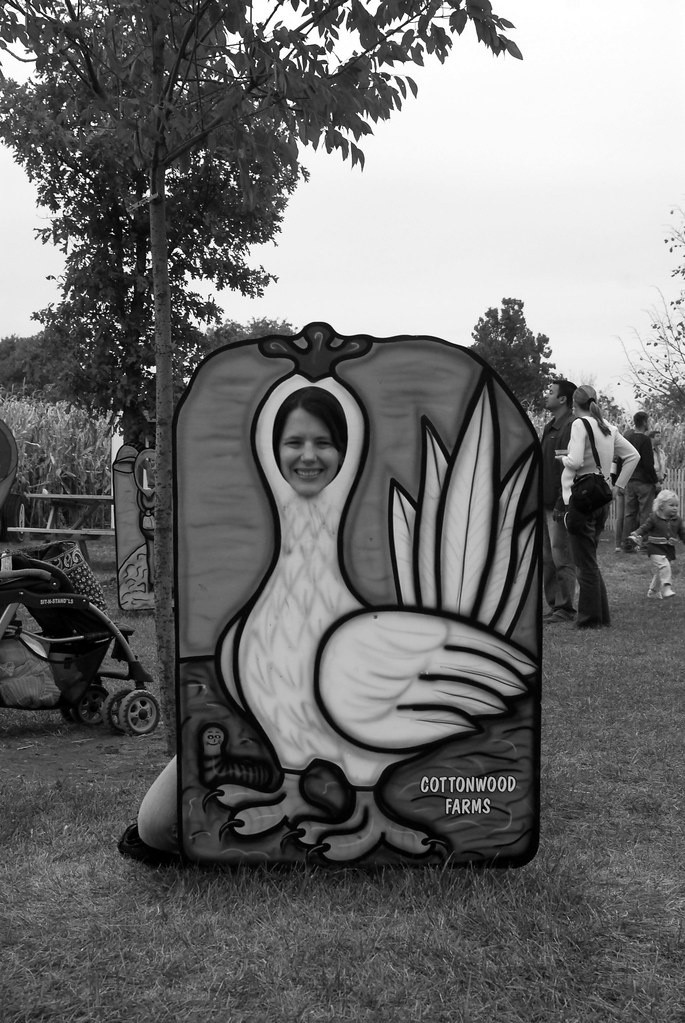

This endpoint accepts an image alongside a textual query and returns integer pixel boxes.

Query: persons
[137,386,348,850]
[610,411,668,553]
[625,489,685,600]
[540,380,641,630]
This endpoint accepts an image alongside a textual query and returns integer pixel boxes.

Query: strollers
[0,540,161,736]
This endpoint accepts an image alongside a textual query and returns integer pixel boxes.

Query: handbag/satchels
[571,473,613,514]
[13,540,108,617]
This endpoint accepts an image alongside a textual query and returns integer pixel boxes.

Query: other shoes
[564,623,610,630]
[663,586,676,597]
[543,612,576,623]
[647,590,663,599]
